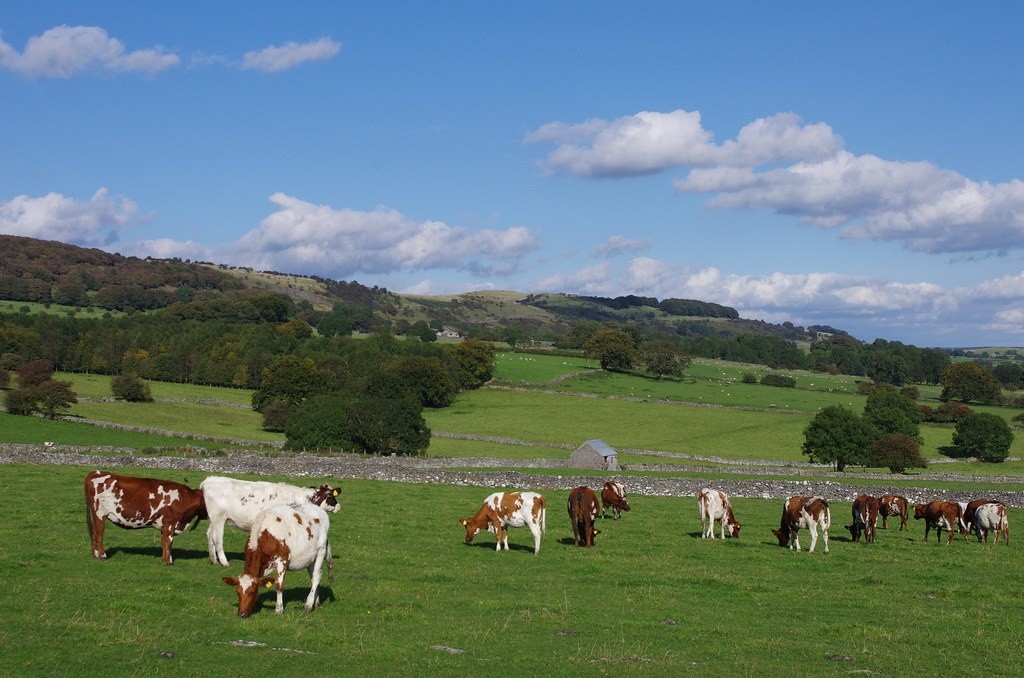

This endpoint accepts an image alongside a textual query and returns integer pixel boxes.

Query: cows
[876,494,909,532]
[601,480,631,521]
[459,491,546,556]
[770,495,831,554]
[843,495,880,545]
[696,488,743,540]
[82,469,343,619]
[567,485,602,547]
[911,499,1010,546]
